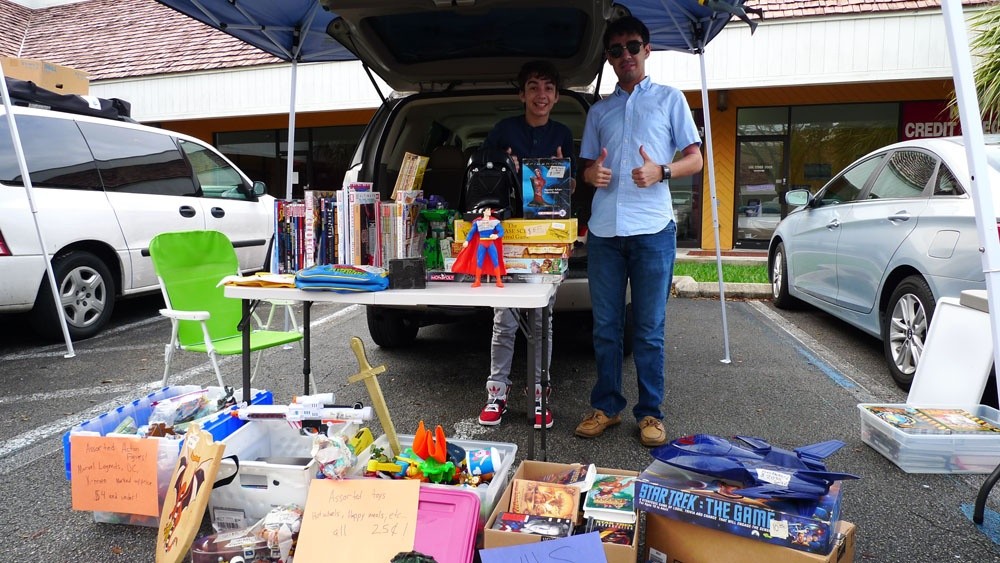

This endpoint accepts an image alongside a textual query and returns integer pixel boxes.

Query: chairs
[149,231,319,397]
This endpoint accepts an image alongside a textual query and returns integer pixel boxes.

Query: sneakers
[478,380,510,425]
[525,383,554,428]
[637,415,666,446]
[576,409,622,438]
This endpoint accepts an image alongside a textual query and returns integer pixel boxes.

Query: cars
[736,202,786,242]
[667,190,703,226]
[764,131,1000,392]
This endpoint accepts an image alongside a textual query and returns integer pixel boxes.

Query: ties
[606,40,649,58]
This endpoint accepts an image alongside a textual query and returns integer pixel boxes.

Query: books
[391,153,430,200]
[863,407,1000,472]
[273,183,424,273]
[492,463,636,545]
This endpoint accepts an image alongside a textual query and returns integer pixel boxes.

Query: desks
[223,279,558,462]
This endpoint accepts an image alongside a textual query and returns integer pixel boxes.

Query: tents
[161,0,762,364]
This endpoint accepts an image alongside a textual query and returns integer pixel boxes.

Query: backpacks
[294,263,390,291]
[458,148,522,217]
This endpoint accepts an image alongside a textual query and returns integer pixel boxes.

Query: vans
[0,106,277,342]
[315,1,633,349]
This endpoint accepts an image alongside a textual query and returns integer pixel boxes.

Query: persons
[477,60,578,429]
[575,15,703,449]
[492,515,563,536]
[416,202,462,271]
[451,208,507,287]
[529,169,546,205]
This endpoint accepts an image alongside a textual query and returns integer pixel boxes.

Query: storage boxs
[0,57,90,95]
[389,258,427,290]
[64,384,855,562]
[858,402,1000,475]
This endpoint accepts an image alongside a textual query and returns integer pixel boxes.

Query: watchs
[658,165,671,183]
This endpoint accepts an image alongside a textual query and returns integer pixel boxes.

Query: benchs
[426,142,597,224]
